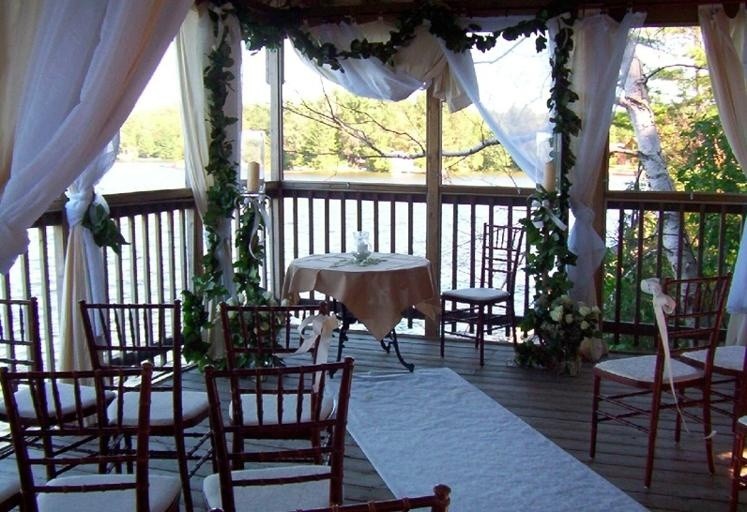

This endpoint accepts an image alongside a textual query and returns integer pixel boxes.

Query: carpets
[325,366,651,511]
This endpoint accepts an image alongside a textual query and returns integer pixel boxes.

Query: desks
[282,251,444,377]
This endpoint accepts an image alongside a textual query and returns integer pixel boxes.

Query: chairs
[0,299,116,481]
[1,360,184,512]
[80,299,217,512]
[202,357,353,512]
[590,270,735,488]
[440,222,524,366]
[1,461,41,512]
[300,483,450,511]
[674,344,747,443]
[728,414,747,511]
[222,302,332,468]
